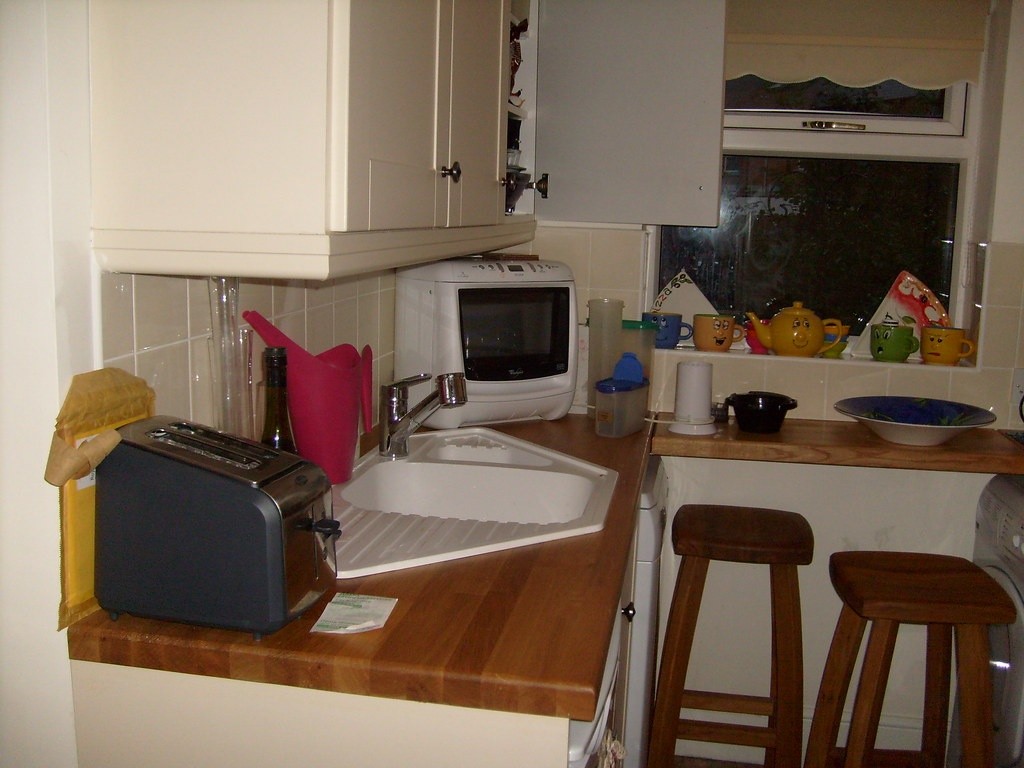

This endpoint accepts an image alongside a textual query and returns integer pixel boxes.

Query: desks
[652,411,1024,474]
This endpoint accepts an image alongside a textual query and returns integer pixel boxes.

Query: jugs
[241,310,373,485]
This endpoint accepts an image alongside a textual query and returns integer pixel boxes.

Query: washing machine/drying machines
[945,473,1024,768]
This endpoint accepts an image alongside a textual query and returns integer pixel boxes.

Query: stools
[642,502,813,768]
[803,551,1017,767]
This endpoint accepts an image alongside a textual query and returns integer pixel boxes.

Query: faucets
[377,370,468,456]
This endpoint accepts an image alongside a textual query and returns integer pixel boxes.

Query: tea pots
[746,301,843,357]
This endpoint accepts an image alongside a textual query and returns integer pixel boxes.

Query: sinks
[336,425,619,527]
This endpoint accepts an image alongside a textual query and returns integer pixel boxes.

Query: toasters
[94,417,341,641]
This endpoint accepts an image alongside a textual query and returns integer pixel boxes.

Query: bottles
[260,345,300,455]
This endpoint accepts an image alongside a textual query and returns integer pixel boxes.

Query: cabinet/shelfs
[84,0,733,279]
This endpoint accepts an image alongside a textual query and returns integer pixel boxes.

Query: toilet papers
[674,361,713,419]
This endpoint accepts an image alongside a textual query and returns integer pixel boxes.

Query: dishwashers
[622,454,668,766]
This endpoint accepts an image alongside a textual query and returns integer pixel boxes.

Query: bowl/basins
[506,173,531,212]
[835,396,998,446]
[726,391,798,434]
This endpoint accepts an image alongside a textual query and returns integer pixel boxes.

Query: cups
[744,320,771,354]
[824,326,850,358]
[507,149,521,173]
[693,314,744,352]
[921,326,975,365]
[871,324,919,362]
[587,299,623,417]
[642,311,693,350]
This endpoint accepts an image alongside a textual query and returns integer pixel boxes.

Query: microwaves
[392,258,578,429]
